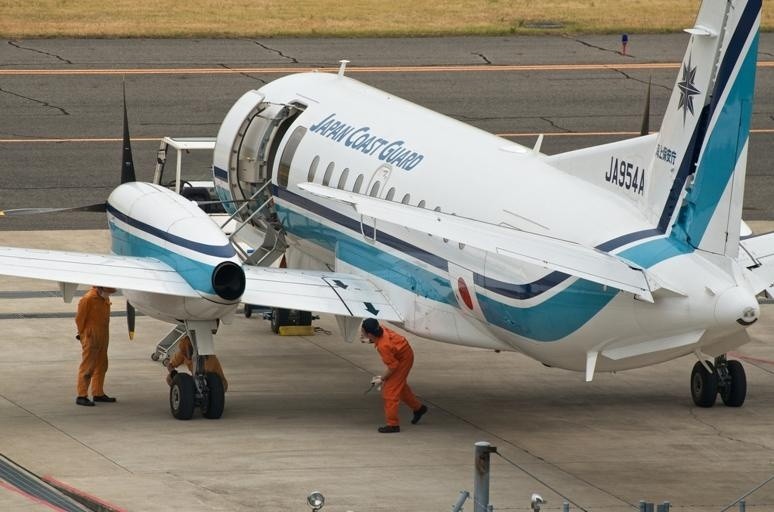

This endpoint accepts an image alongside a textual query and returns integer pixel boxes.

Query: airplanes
[0,0,773,422]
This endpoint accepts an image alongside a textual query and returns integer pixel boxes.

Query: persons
[360,318,428,434]
[75,285,116,407]
[166,335,228,394]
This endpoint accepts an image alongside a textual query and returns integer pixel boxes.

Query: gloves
[371,376,385,392]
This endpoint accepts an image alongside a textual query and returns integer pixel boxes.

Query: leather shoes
[411,405,426,424]
[378,425,400,432]
[93,395,115,402]
[77,397,94,406]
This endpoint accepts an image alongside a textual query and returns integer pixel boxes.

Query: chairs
[180,187,211,213]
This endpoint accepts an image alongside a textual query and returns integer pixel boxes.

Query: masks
[98,289,111,299]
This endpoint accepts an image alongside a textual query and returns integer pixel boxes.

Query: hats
[179,337,192,352]
[364,318,382,336]
[103,287,115,293]
